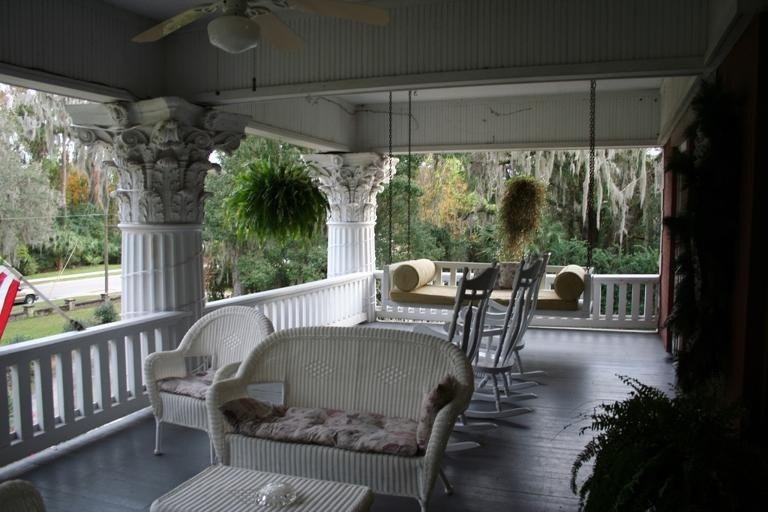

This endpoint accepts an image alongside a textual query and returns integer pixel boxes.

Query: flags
[0,265,21,340]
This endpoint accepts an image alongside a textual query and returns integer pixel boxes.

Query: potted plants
[497,175,548,289]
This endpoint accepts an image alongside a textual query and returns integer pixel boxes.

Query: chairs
[144,306,274,455]
[412,252,551,453]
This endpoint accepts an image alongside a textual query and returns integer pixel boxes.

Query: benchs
[205,327,474,512]
[382,260,595,318]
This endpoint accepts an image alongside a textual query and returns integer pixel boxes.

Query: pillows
[394,259,436,292]
[554,264,585,301]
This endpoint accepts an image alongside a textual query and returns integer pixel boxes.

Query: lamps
[208,16,259,95]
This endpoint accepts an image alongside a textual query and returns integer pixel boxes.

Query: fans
[129,0,390,49]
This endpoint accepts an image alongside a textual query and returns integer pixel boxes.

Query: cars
[14,286,39,304]
